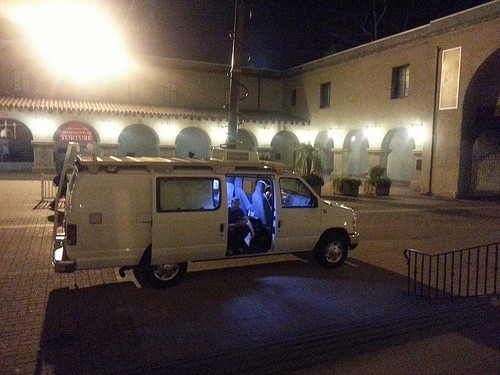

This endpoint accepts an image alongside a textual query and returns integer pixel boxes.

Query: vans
[47,141,358,289]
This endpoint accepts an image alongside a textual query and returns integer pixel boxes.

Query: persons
[227,197,255,255]
[49,170,69,208]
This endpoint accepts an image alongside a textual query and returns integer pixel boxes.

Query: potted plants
[332,177,363,196]
[290,142,324,198]
[364,164,392,196]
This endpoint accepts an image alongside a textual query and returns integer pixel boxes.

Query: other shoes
[227,248,244,254]
[49,204,54,208]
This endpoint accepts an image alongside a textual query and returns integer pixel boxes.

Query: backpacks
[52,174,60,186]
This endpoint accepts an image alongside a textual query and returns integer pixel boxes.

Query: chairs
[234,176,251,216]
[252,180,273,226]
